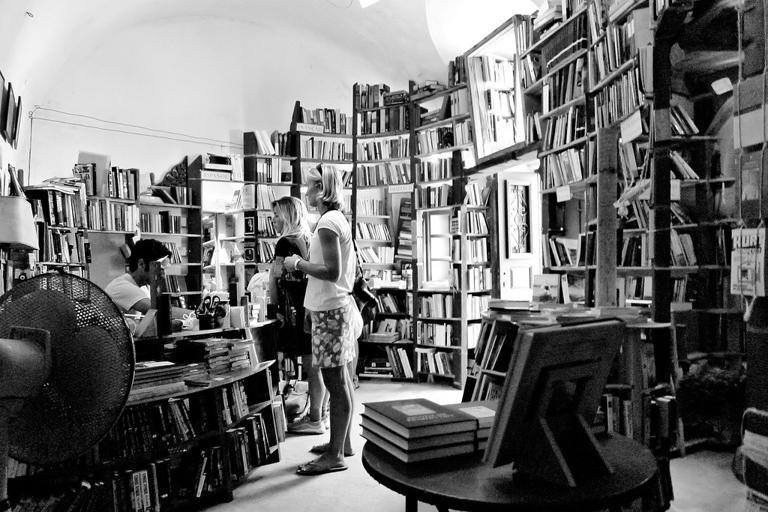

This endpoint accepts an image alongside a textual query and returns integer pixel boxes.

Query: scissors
[204,296,220,313]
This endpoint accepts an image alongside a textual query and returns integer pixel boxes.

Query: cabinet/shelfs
[0,0,767,512]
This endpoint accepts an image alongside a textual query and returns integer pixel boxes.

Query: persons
[268,162,362,475]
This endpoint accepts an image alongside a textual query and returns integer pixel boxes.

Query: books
[516,1,767,450]
[481,316,625,469]
[359,372,500,464]
[354,55,539,380]
[241,103,356,380]
[1,150,287,510]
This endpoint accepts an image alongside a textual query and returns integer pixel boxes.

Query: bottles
[156,293,172,335]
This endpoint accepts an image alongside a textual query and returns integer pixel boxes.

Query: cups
[230,305,245,329]
[199,312,215,330]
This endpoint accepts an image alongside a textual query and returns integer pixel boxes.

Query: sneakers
[287,416,326,435]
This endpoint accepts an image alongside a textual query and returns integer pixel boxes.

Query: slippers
[311,443,356,457]
[295,461,349,476]
